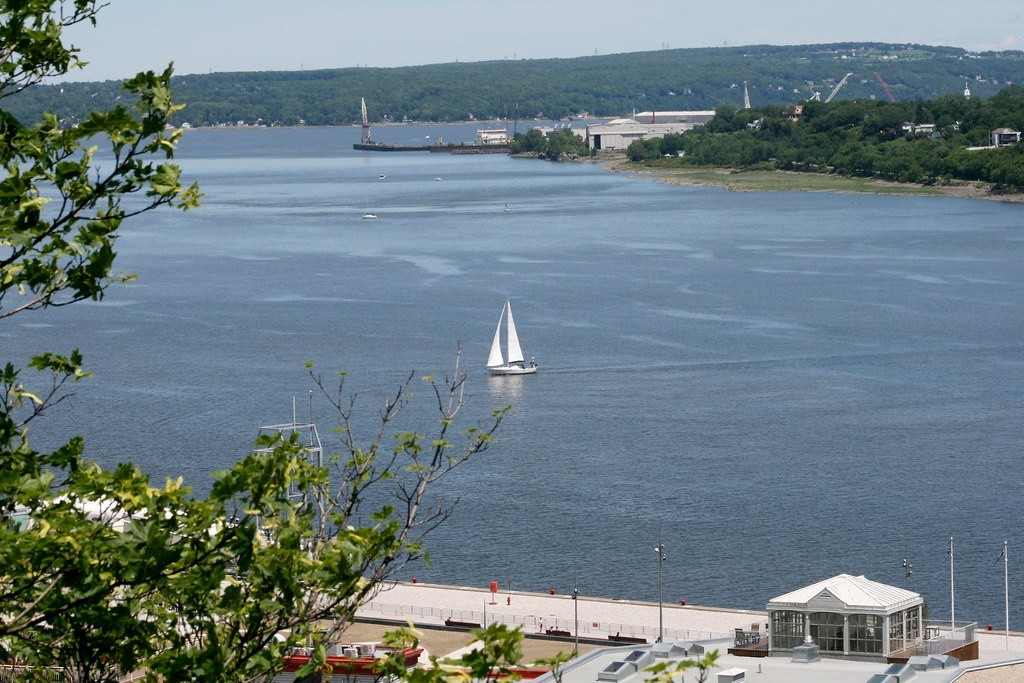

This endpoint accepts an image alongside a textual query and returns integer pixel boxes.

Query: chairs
[735,623,759,648]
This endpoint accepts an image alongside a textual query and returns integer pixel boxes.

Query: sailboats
[361,97,370,128]
[485,299,539,375]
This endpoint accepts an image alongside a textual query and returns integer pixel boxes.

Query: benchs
[546,630,572,636]
[445,621,481,628]
[608,636,646,645]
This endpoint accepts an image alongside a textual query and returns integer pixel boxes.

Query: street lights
[654,535,667,643]
[902,545,913,591]
[571,588,580,658]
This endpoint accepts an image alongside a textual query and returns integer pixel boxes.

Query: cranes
[873,72,897,103]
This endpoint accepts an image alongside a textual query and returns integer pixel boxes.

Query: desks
[925,626,939,639]
[744,631,759,644]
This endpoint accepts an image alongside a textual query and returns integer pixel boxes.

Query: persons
[538,617,560,633]
[447,617,452,621]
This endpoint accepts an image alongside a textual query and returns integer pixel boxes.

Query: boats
[362,213,377,219]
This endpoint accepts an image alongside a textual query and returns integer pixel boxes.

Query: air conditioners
[361,644,375,655]
[293,648,305,655]
[344,648,358,658]
